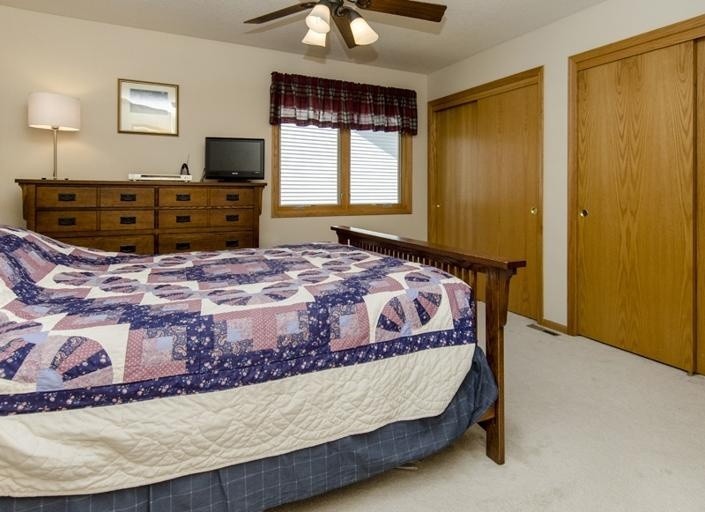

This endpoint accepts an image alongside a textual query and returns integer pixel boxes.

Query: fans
[244,0,448,49]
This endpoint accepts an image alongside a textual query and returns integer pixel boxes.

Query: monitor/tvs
[204,137,265,183]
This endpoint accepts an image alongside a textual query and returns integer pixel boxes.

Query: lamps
[301,0,379,47]
[27,92,80,181]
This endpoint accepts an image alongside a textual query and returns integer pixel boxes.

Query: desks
[15,179,268,255]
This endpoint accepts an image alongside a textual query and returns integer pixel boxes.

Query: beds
[0,225,527,512]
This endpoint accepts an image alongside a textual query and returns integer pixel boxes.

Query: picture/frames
[117,79,179,137]
